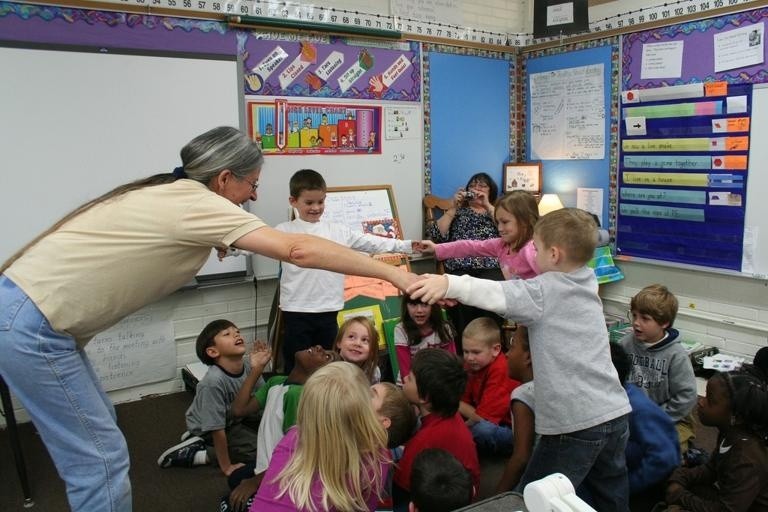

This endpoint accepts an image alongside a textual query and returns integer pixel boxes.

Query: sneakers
[157,432,205,467]
[686,447,710,466]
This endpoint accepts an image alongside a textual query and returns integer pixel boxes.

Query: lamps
[537,194,565,216]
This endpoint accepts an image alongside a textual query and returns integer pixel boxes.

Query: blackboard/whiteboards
[1,46,247,285]
[237,96,425,286]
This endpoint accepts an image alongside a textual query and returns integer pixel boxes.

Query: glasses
[240,179,259,193]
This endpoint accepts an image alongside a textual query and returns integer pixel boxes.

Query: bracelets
[446,210,456,218]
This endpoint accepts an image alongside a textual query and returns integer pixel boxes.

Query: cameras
[463,191,476,201]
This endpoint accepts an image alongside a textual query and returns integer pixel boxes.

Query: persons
[157,320,268,468]
[215,168,432,376]
[607,340,680,510]
[411,190,540,326]
[407,206,631,511]
[656,370,767,512]
[426,172,508,351]
[0,126,428,512]
[220,290,536,512]
[619,284,697,462]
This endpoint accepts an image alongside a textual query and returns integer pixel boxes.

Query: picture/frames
[504,164,542,192]
